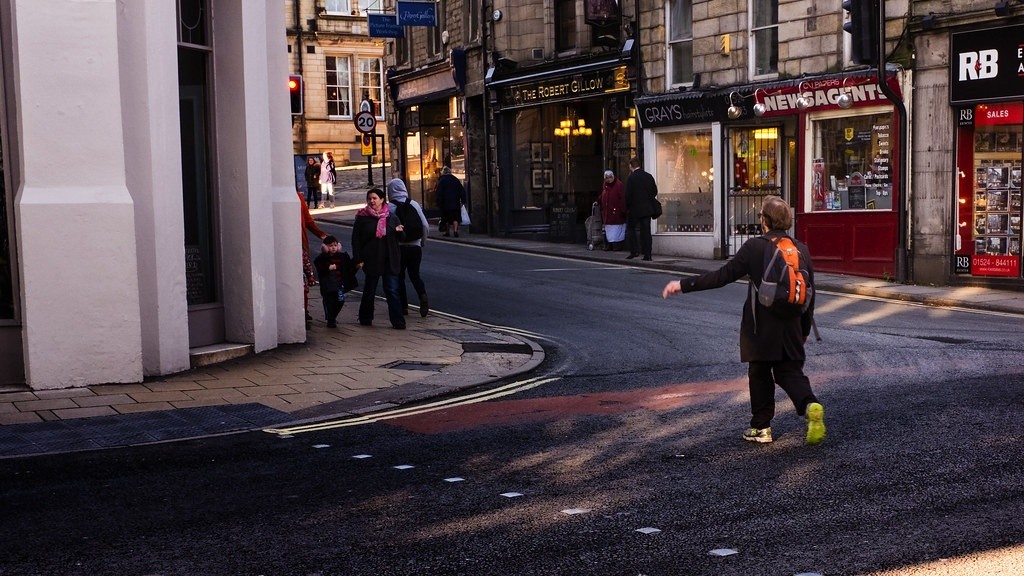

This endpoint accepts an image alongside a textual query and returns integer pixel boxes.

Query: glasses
[758,213,763,220]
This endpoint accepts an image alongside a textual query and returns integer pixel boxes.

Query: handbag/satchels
[459,204,472,227]
[649,197,663,219]
[438,219,447,232]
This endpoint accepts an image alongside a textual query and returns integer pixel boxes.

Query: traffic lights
[289,74,304,116]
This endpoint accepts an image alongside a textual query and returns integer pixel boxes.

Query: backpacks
[753,232,813,319]
[389,198,423,243]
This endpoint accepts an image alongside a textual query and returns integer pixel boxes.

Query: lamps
[498,57,519,68]
[598,34,617,49]
[753,88,782,117]
[728,91,764,120]
[554,106,592,137]
[837,77,857,109]
[755,128,777,139]
[796,81,824,109]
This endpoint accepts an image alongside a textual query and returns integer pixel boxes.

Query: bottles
[814,174,823,209]
[825,182,846,210]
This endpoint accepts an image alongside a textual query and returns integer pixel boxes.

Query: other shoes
[318,204,325,209]
[314,204,318,209]
[641,255,652,261]
[403,308,409,315]
[392,320,406,330]
[360,318,373,326]
[305,312,312,320]
[626,250,639,259]
[613,245,622,252]
[327,317,338,328]
[420,293,428,317]
[605,245,612,250]
[454,232,458,237]
[330,204,336,208]
[443,233,450,237]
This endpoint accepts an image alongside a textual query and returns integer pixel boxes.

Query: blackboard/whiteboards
[550,206,578,242]
[848,185,867,209]
[185,245,207,298]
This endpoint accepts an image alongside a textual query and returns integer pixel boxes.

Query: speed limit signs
[354,110,377,133]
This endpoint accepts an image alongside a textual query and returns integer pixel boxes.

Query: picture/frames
[530,167,554,191]
[529,140,553,164]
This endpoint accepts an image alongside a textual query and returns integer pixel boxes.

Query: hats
[442,166,451,175]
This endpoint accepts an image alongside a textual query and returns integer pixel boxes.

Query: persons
[595,170,626,250]
[305,151,336,208]
[387,177,430,318]
[297,192,328,320]
[832,192,841,208]
[352,188,406,330]
[314,235,363,328]
[624,157,658,261]
[436,166,466,238]
[663,196,825,444]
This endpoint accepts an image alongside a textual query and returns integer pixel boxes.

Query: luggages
[584,201,605,250]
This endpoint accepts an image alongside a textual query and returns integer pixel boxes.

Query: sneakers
[804,402,826,446]
[743,427,772,443]
